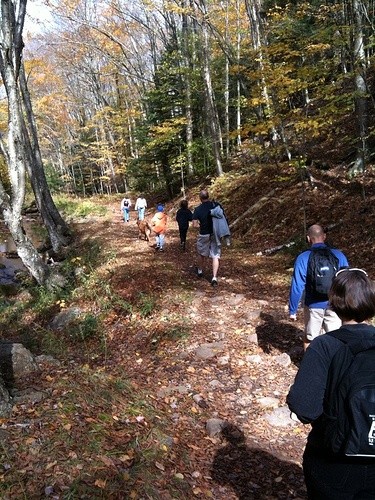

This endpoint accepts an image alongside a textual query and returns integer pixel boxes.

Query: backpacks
[306,246,338,305]
[327,326,375,457]
[124,201,128,206]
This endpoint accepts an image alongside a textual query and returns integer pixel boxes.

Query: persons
[176,200,193,252]
[192,190,226,286]
[289,223,349,351]
[150,205,168,251]
[135,195,147,221]
[121,195,131,222]
[286,267,375,500]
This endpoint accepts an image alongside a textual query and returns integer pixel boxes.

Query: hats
[158,206,163,211]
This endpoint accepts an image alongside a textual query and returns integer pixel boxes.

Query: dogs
[136,219,152,242]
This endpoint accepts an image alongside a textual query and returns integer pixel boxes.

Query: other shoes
[211,277,217,285]
[197,268,202,276]
[156,244,160,248]
[160,248,163,252]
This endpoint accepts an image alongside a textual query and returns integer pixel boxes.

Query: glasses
[333,265,368,278]
